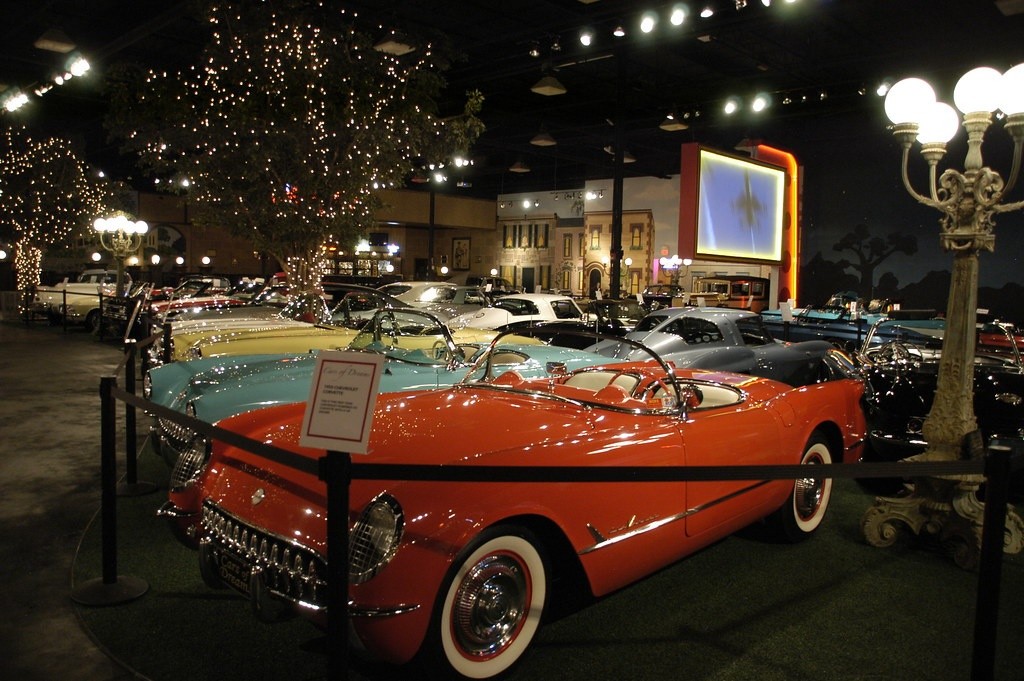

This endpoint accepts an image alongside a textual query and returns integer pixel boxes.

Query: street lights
[427,152,469,283]
[94,216,148,301]
[863,63,1024,570]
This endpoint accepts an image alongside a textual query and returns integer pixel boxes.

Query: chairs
[444,344,482,363]
[492,349,530,363]
[641,378,743,409]
[562,369,641,399]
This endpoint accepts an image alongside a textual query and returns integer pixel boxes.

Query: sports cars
[155,328,867,681]
[32,271,1024,488]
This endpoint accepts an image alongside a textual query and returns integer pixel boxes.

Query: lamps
[2,42,95,118]
[665,82,894,119]
[522,0,804,60]
